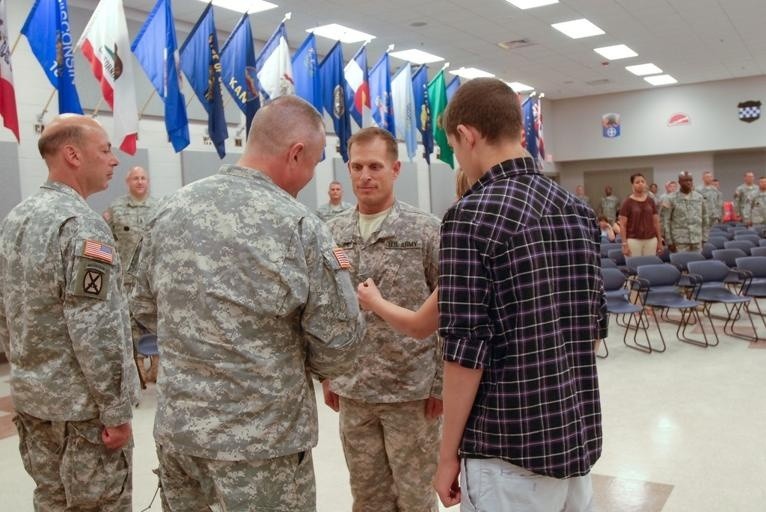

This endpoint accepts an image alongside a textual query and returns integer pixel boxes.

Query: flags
[131,4,192,154]
[218,10,261,140]
[178,3,229,159]
[291,32,461,170]
[255,22,297,103]
[1,6,20,144]
[77,2,140,154]
[20,3,85,117]
[520,96,545,173]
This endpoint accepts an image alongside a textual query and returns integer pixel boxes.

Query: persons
[316,179,349,219]
[120,92,353,511]
[575,156,764,316]
[311,126,452,511]
[105,167,158,371]
[450,163,470,202]
[432,74,608,510]
[1,114,142,511]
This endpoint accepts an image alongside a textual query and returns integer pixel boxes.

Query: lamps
[593,222,766,359]
